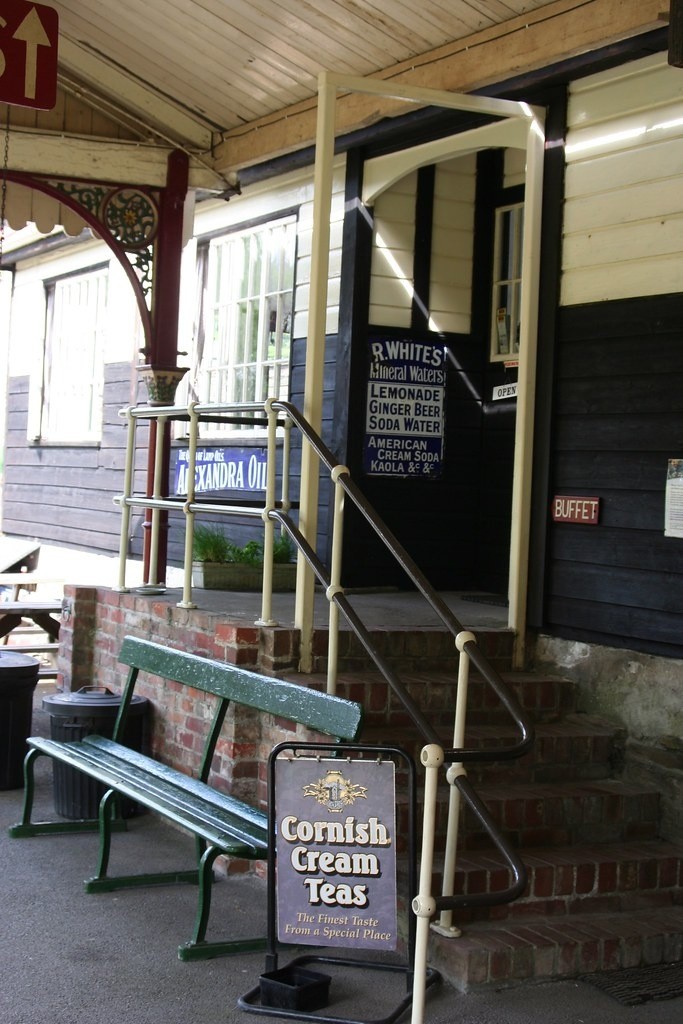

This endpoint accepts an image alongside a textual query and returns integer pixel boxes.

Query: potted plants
[190,520,298,593]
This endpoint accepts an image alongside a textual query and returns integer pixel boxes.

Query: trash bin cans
[0,651,41,793]
[40,683,150,820]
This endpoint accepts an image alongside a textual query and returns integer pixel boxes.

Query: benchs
[7,636,363,961]
